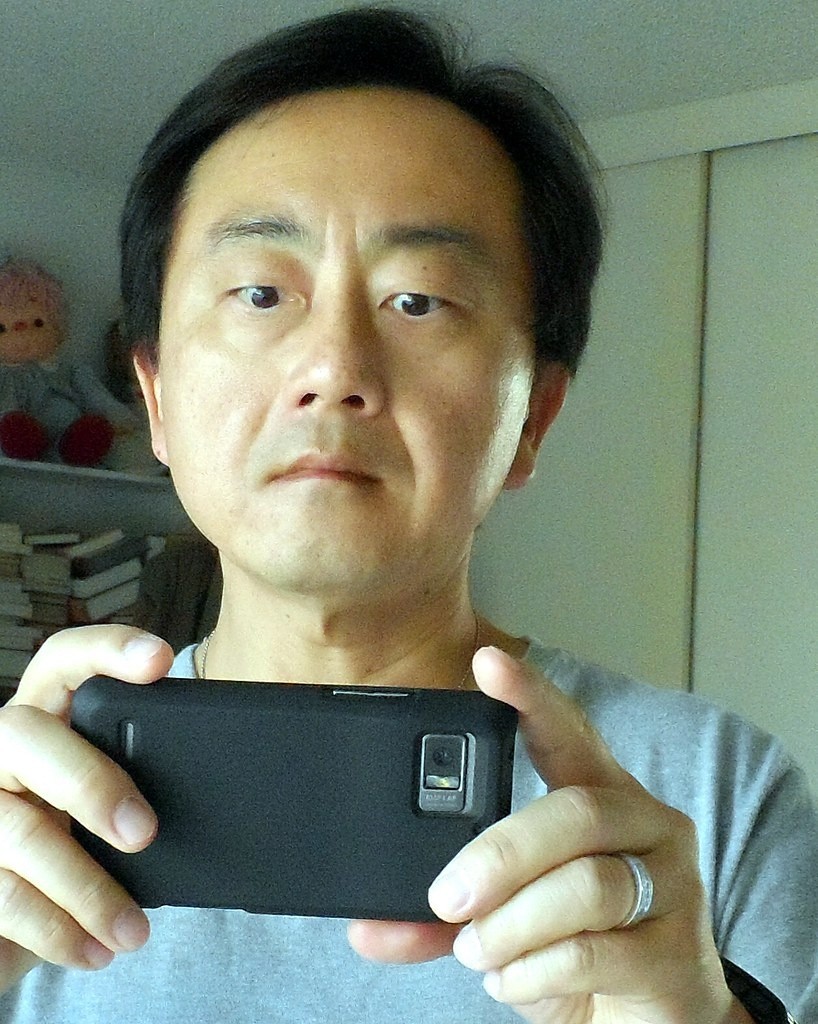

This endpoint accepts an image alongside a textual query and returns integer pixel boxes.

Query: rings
[612,851,653,930]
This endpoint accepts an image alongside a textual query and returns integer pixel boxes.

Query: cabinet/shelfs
[463,78,818,813]
[0,453,216,699]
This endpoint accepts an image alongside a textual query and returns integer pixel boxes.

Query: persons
[0,5,818,1024]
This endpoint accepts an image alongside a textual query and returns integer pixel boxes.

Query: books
[0,522,204,680]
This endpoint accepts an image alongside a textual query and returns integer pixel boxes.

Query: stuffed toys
[0,256,164,473]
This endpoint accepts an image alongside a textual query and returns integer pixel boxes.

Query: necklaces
[200,607,480,691]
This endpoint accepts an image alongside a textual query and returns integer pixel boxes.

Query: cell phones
[69,676,521,922]
